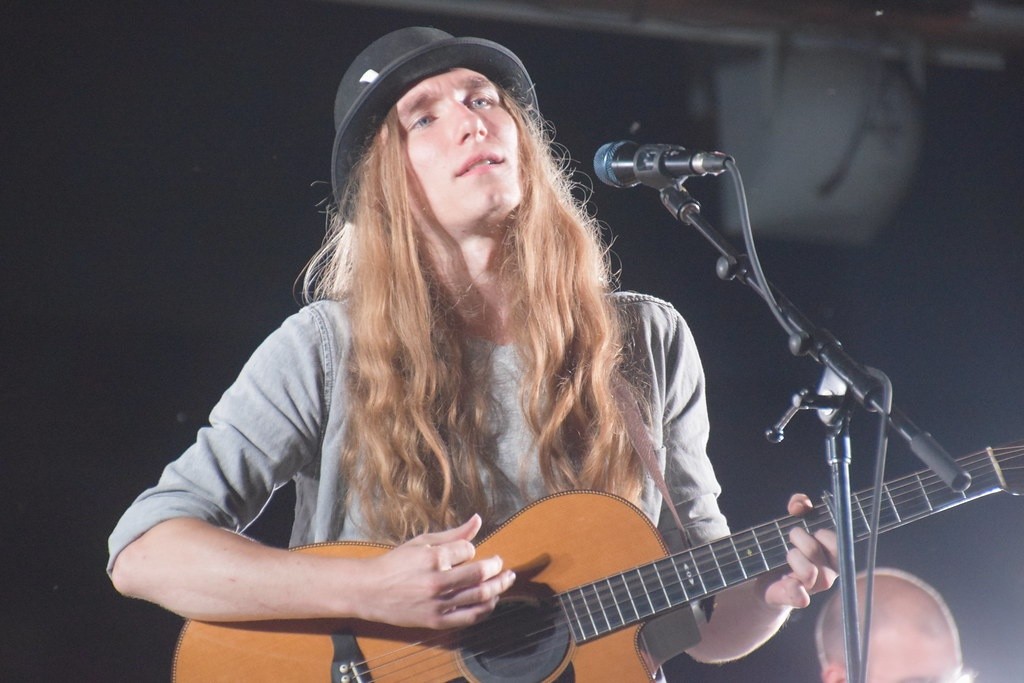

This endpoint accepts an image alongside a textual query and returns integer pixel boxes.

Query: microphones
[593,140,736,190]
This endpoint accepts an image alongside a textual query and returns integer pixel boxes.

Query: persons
[106,30,840,682]
[816,566,967,683]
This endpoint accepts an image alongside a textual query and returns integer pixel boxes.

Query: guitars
[170,441,1023,683]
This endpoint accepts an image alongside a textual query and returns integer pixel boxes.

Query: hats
[330,25,540,224]
